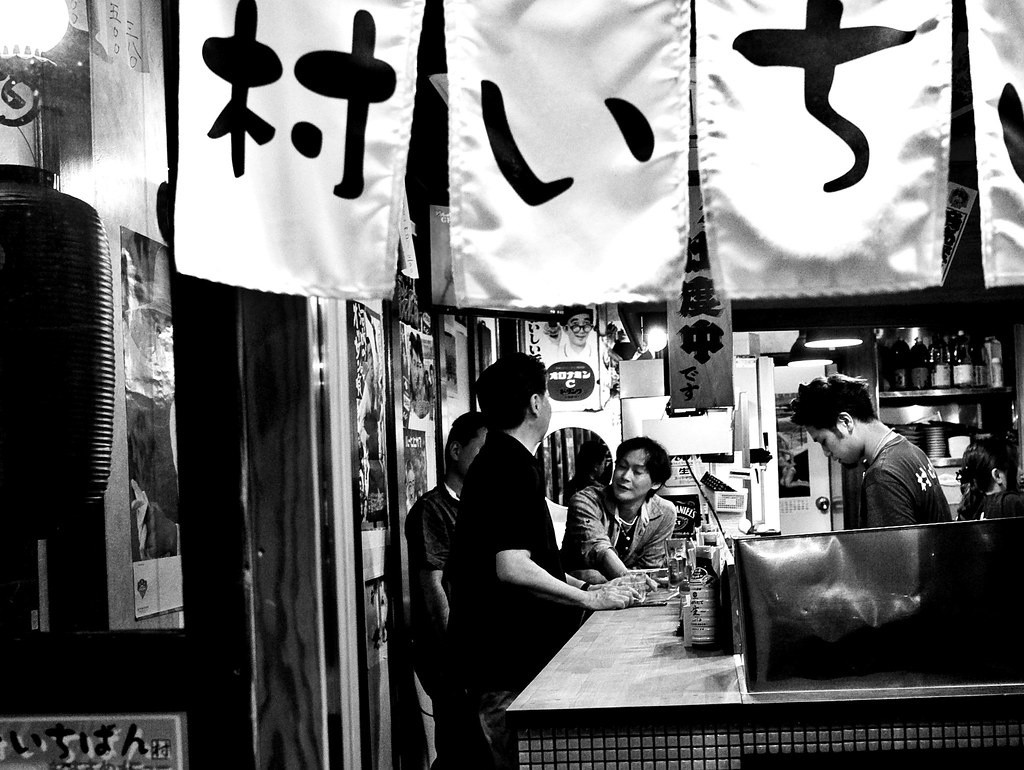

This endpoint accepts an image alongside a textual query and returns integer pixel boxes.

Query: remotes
[700,471,737,492]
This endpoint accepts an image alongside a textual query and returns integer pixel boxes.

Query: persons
[355,303,610,532]
[788,375,951,528]
[446,352,646,770]
[559,436,677,624]
[404,412,488,770]
[956,435,1024,521]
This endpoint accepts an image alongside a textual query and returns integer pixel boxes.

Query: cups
[620,571,646,604]
[664,538,687,593]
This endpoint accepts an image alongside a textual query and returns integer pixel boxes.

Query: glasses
[568,323,594,334]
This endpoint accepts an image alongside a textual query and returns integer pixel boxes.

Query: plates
[897,430,922,450]
[925,424,947,459]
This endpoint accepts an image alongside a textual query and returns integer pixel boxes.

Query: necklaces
[870,427,895,466]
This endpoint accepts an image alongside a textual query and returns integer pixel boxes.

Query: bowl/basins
[949,436,972,457]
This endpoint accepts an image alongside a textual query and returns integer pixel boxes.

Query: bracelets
[580,581,591,591]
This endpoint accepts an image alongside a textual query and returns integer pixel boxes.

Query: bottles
[889,330,1007,391]
[679,578,691,620]
[702,523,716,534]
[703,534,716,547]
[690,547,722,651]
